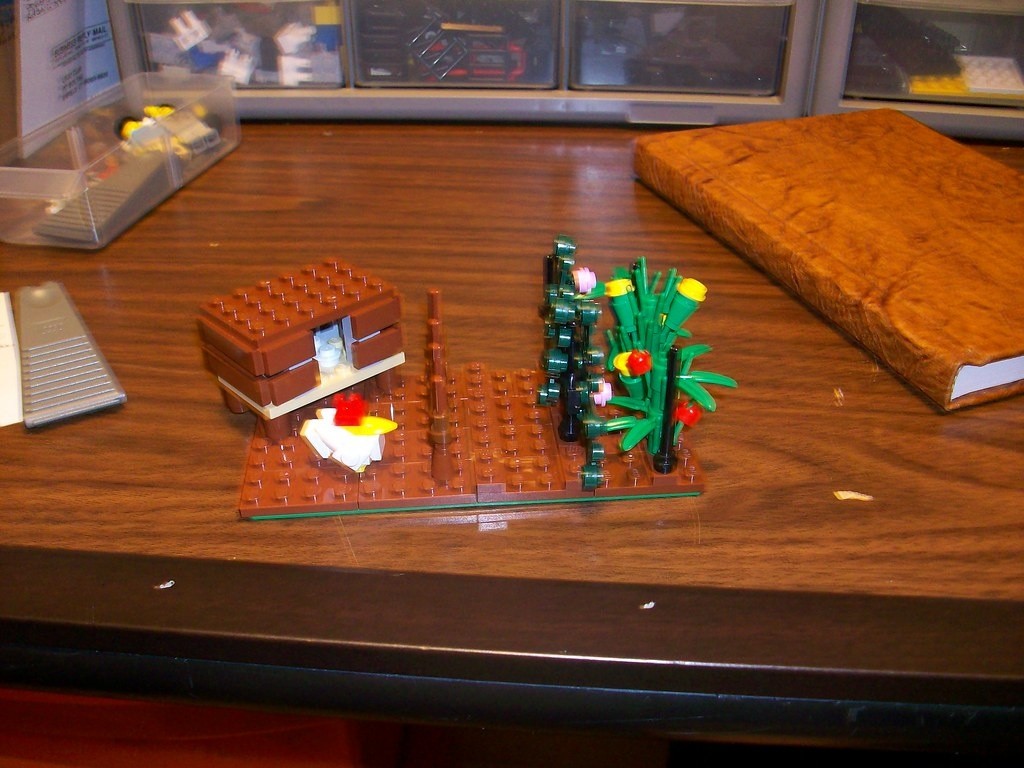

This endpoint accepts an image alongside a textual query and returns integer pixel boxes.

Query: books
[632,108,1024,412]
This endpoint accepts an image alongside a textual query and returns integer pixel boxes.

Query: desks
[0,118,1024,681]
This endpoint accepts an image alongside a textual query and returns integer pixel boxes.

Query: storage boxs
[106,0,349,120]
[0,70,242,254]
[569,0,827,125]
[345,1,568,122]
[806,0,1024,142]
